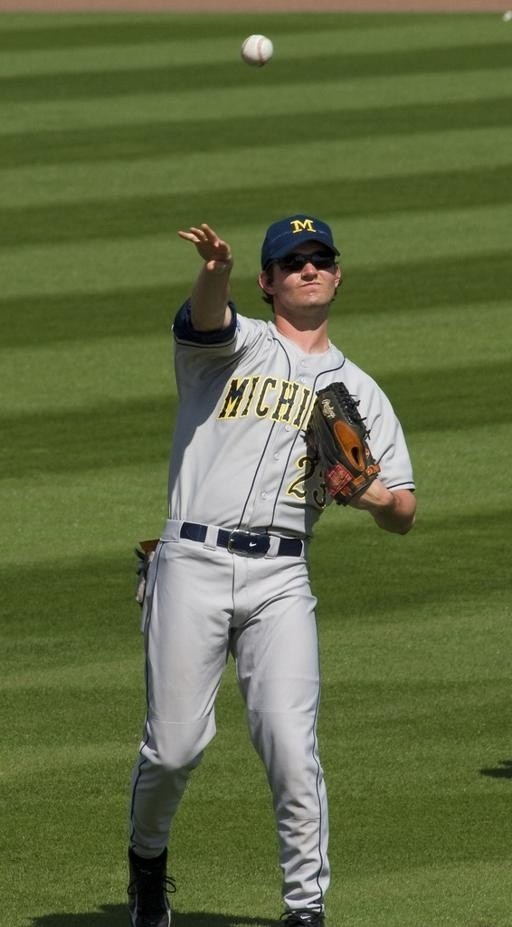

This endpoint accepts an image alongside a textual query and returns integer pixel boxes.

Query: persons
[118,214,422,927]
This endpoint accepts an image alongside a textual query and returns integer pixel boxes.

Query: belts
[180,521,302,560]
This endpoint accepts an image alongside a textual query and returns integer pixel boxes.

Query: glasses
[263,249,336,273]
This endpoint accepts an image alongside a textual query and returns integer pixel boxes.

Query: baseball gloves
[308,382,381,508]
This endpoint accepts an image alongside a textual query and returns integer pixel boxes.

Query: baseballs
[240,33,273,65]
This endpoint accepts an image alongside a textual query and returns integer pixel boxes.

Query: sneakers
[126,844,174,926]
[278,908,325,925]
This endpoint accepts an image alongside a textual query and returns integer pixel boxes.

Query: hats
[260,213,341,272]
